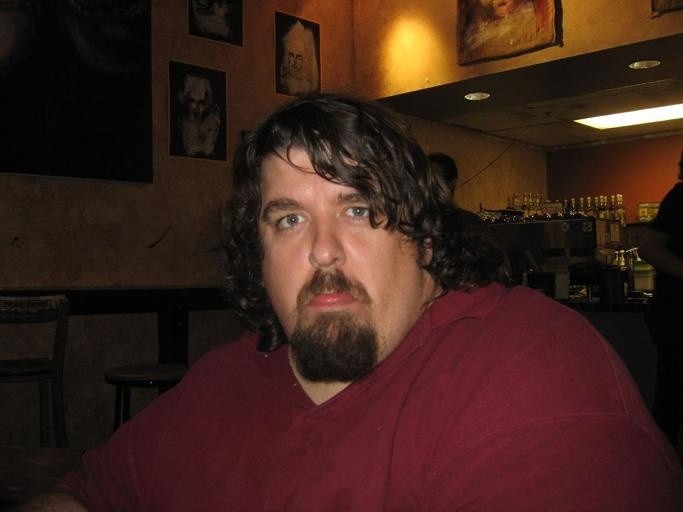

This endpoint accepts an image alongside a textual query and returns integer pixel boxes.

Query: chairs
[0,295,69,449]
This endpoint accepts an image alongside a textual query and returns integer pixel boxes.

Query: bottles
[510,191,627,228]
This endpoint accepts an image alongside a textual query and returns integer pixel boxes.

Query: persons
[626,153,681,455]
[7,88,681,512]
[411,149,492,246]
[174,75,222,156]
[278,19,318,95]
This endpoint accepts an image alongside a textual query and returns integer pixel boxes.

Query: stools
[104,365,181,433]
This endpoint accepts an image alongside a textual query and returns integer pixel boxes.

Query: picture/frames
[186,0,245,50]
[274,9,322,100]
[168,59,228,165]
